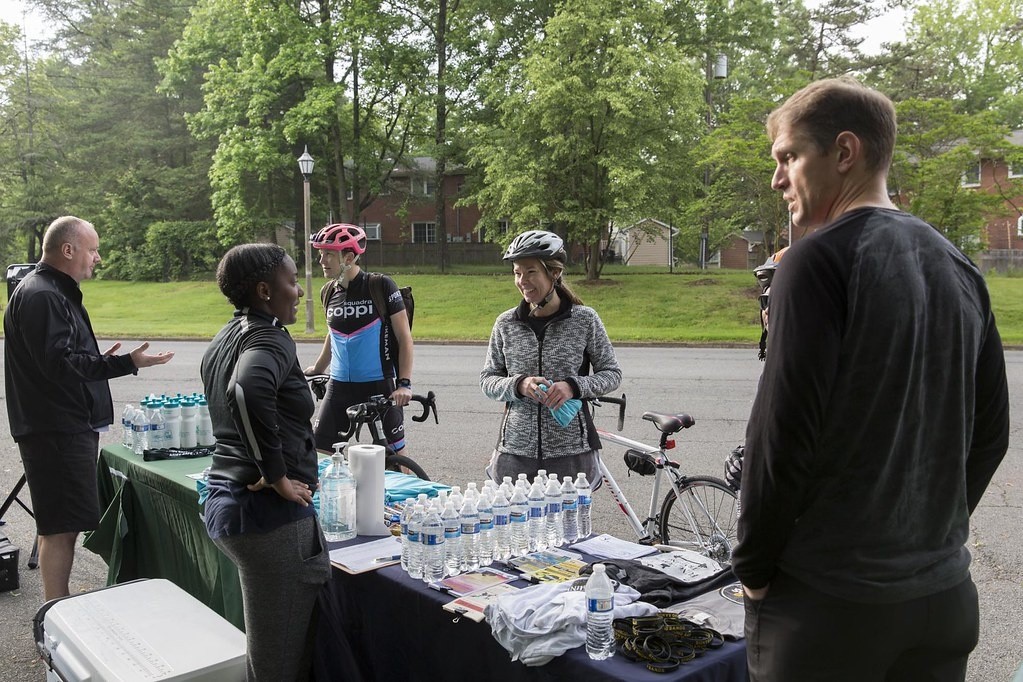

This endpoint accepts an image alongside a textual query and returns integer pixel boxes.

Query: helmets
[312,224,368,255]
[502,230,568,263]
[753,246,790,290]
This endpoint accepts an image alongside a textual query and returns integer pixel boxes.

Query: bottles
[585,564,616,660]
[121,392,216,455]
[401,470,591,581]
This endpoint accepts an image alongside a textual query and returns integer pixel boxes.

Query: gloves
[724,446,745,493]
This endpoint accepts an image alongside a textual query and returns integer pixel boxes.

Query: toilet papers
[348,444,393,536]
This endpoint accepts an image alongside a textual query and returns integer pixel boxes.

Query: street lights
[297,145,315,334]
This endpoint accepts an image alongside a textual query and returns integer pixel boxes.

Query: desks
[326,534,750,682]
[81,443,245,635]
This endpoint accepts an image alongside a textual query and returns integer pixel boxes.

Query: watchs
[396,378,411,386]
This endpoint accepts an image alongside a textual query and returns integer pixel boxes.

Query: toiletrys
[318,442,357,542]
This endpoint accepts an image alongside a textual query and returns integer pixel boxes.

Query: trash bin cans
[6,263,37,304]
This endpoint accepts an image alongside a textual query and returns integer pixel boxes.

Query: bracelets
[398,384,411,389]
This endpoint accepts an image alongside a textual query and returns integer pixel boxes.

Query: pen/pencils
[375,554,401,563]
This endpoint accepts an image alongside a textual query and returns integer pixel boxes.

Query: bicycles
[337,392,439,482]
[587,393,739,570]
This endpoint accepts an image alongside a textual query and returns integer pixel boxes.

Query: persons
[480,230,622,489]
[724,75,1012,682]
[203,243,363,681]
[3,214,176,602]
[301,223,412,476]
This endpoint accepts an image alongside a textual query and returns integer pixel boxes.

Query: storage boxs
[33,577,246,682]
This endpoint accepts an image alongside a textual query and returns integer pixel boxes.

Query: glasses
[757,294,769,311]
